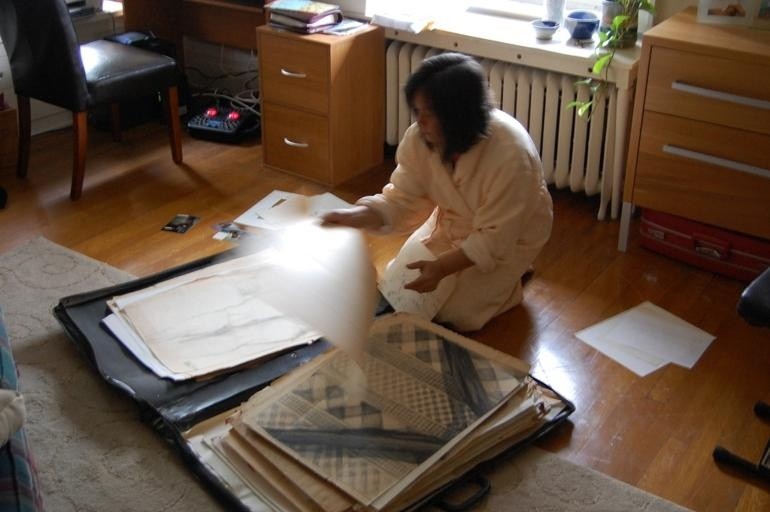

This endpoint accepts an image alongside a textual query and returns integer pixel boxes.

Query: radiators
[384,41,618,224]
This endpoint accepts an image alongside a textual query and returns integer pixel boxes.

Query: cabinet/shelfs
[613,0,769,259]
[257,18,386,188]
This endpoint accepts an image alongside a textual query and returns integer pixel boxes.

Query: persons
[319,52,554,334]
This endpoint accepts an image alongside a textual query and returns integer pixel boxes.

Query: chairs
[0,1,184,200]
[712,267,769,490]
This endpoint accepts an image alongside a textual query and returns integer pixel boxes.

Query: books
[263,8,343,35]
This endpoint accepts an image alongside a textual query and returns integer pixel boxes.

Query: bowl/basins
[532,19,560,39]
[564,10,600,40]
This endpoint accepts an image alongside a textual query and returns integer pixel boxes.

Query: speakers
[104,32,159,132]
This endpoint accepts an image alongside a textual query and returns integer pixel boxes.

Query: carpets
[0,233,693,512]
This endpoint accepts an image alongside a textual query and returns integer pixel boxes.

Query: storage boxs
[639,210,770,285]
